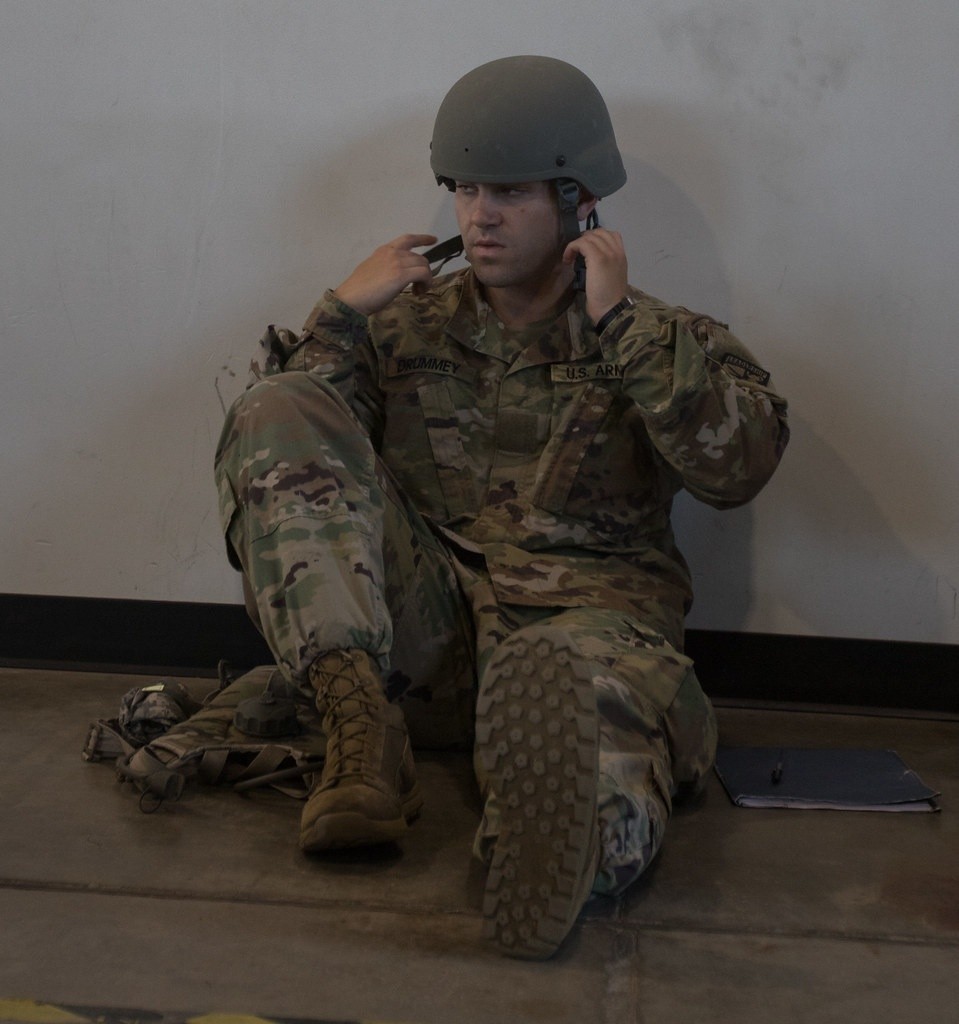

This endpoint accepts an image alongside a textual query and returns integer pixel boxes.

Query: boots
[296,646,424,855]
[473,627,599,962]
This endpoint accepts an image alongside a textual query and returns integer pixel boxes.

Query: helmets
[430,55,627,196]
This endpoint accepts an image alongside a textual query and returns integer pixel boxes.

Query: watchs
[594,295,643,337]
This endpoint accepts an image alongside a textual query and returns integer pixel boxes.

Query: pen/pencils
[772,761,784,782]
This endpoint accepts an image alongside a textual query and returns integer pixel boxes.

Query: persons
[212,54,789,958]
